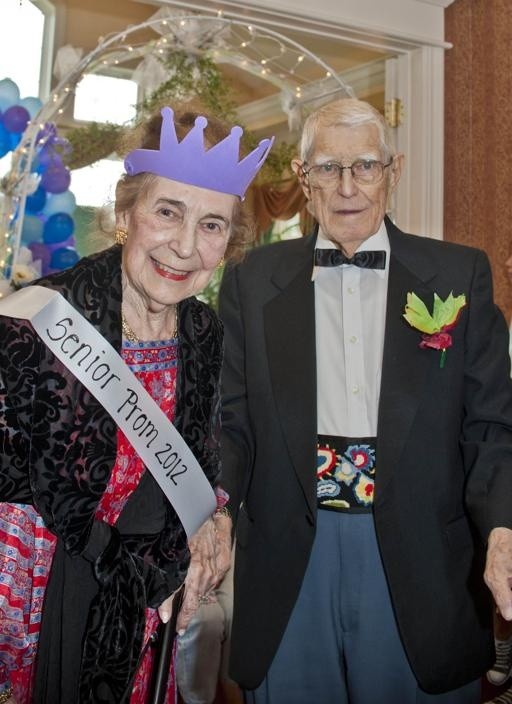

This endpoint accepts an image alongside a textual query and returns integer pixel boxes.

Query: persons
[1,104,277,703]
[211,94,511,701]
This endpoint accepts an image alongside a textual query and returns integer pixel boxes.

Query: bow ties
[315,249,387,270]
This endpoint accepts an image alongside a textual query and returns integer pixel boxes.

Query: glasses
[300,160,392,186]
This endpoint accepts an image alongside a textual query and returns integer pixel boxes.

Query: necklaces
[115,305,179,344]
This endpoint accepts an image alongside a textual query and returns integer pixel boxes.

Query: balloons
[1,75,82,280]
